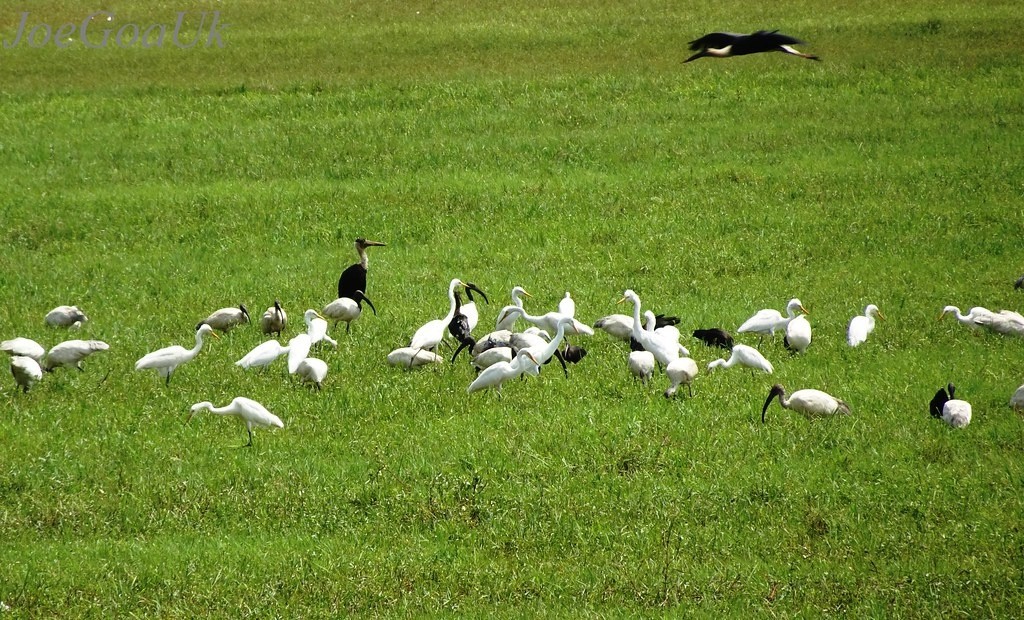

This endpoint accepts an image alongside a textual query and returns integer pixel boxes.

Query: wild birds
[197,303,334,392]
[762,383,847,427]
[594,290,697,402]
[682,28,821,66]
[938,305,1024,341]
[927,382,974,428]
[691,298,812,378]
[45,306,87,331]
[388,278,594,400]
[0,336,111,391]
[848,304,887,349]
[132,323,219,390]
[184,395,282,448]
[325,236,388,333]
